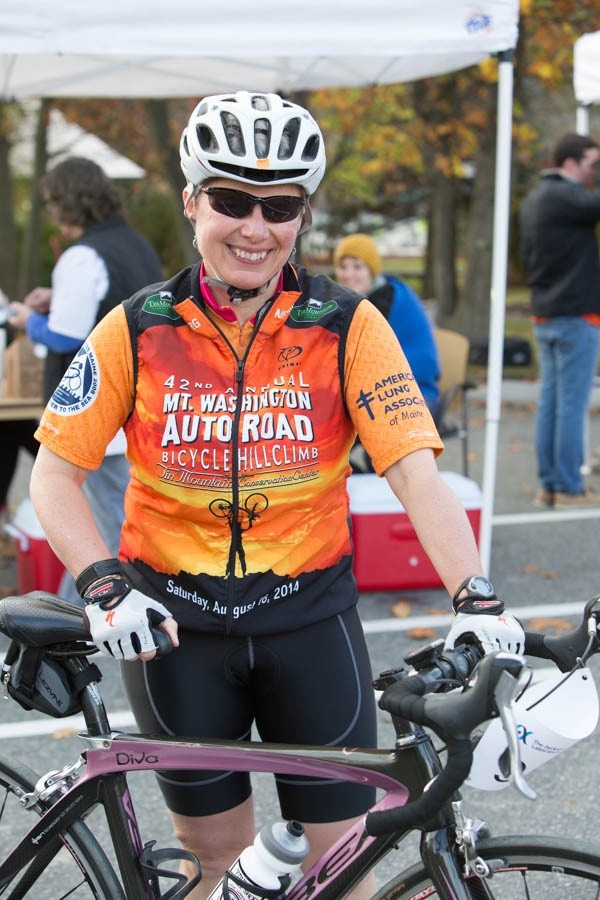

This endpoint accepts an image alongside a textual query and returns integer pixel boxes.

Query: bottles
[206,820,307,900]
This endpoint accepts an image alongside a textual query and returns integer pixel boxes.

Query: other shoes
[533,488,600,510]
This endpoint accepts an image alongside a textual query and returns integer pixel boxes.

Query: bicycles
[1,587,600,900]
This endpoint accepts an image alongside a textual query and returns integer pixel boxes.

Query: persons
[518,129,600,508]
[29,92,526,900]
[9,158,168,610]
[332,233,441,472]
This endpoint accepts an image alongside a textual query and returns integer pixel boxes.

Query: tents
[0,0,517,578]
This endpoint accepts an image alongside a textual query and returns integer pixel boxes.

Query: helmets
[178,92,327,196]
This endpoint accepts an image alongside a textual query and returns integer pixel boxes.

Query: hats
[334,234,381,280]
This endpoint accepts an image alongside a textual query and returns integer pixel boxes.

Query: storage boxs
[348,474,482,589]
[5,500,67,598]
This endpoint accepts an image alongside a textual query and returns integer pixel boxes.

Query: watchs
[451,575,498,615]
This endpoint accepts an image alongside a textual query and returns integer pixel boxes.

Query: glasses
[197,188,306,223]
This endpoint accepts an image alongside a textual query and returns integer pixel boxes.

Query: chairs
[429,329,475,476]
